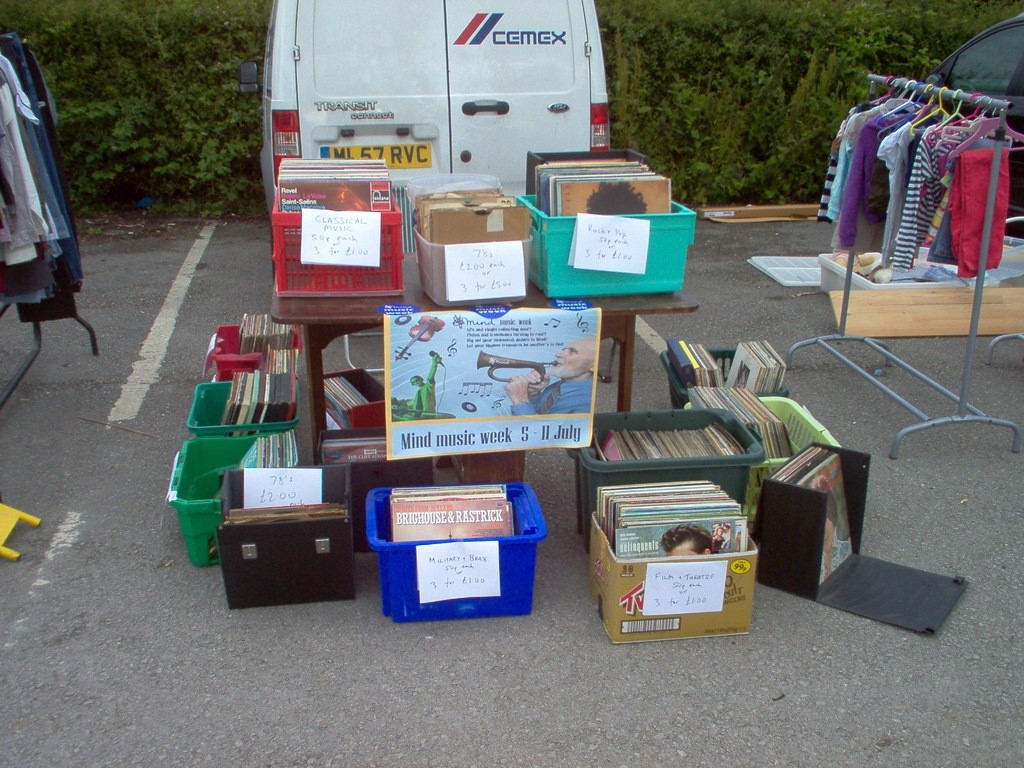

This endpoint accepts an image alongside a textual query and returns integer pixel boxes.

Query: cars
[903,13,1024,240]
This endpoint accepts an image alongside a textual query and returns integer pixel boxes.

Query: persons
[505,337,597,416]
[406,354,442,415]
[812,476,851,582]
[721,524,731,549]
[662,525,712,555]
[713,527,724,550]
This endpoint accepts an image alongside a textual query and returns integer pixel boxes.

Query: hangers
[847,75,1024,158]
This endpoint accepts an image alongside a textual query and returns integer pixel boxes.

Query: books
[666,337,787,392]
[415,188,532,245]
[209,311,301,561]
[687,385,794,458]
[597,479,749,559]
[323,376,370,430]
[534,158,671,217]
[592,424,747,463]
[322,436,387,463]
[279,158,392,215]
[772,446,853,584]
[387,484,512,541]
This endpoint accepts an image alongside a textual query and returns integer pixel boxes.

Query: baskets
[682,395,841,522]
[270,186,405,299]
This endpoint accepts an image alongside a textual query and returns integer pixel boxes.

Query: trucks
[239,0,611,285]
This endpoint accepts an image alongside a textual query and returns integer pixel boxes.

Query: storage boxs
[515,194,697,300]
[658,349,789,408]
[818,237,1024,296]
[412,224,534,308]
[187,382,301,436]
[566,407,766,555]
[272,193,407,298]
[212,325,302,380]
[321,368,384,432]
[170,431,277,568]
[684,397,842,522]
[365,482,547,623]
[588,510,759,644]
